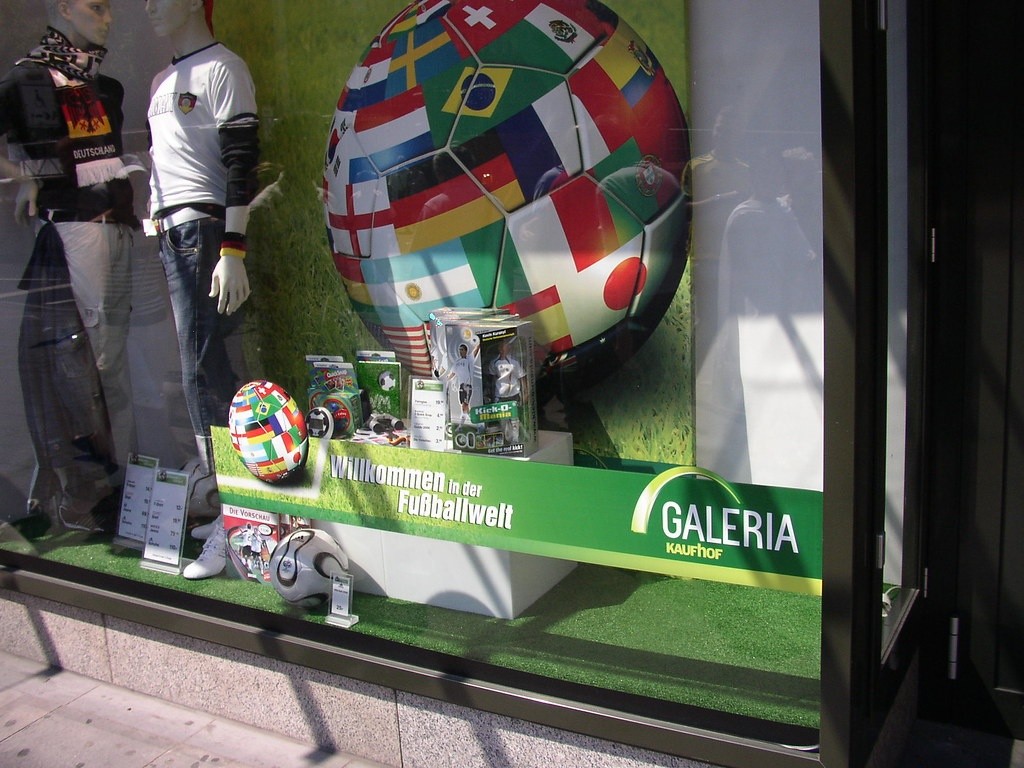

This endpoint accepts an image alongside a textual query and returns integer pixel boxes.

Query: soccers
[228,377,305,483]
[322,2,690,394]
[272,527,346,610]
[377,370,395,392]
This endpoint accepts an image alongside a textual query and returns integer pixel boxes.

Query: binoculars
[366,412,404,433]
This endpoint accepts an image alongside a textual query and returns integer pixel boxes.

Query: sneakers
[183,521,226,579]
[190,513,223,539]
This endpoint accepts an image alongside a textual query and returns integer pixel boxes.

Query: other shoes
[476,425,485,435]
[455,427,463,432]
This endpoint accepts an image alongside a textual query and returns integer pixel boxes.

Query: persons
[446,330,485,435]
[488,336,527,446]
[241,106,857,432]
[0,0,145,532]
[138,0,262,581]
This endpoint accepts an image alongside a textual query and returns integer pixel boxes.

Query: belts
[142,208,211,238]
[38,208,140,231]
